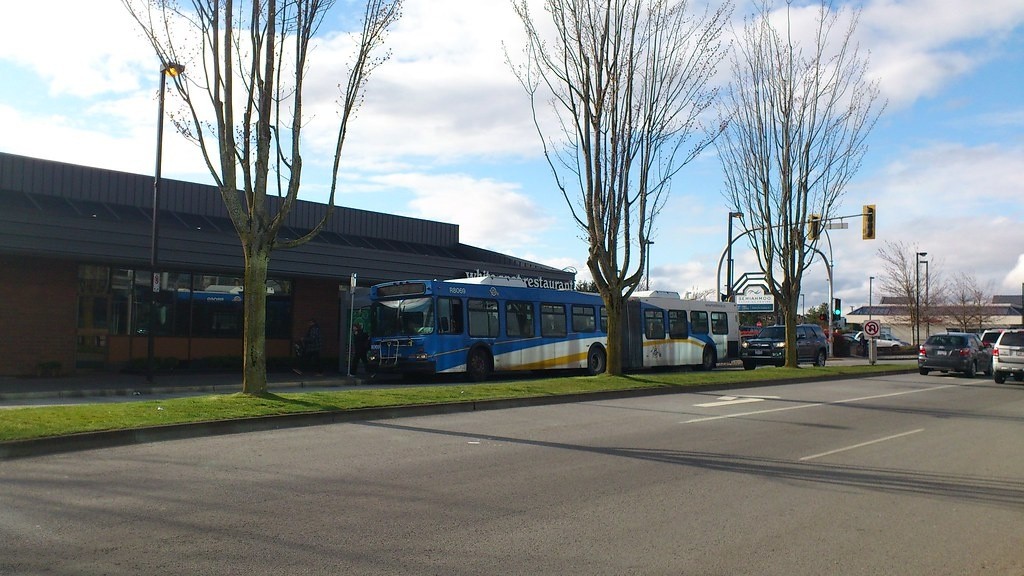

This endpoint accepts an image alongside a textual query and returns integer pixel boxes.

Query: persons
[860,333,869,358]
[289,318,324,377]
[350,325,376,378]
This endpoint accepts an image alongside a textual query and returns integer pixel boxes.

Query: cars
[980,328,1004,347]
[843,329,910,349]
[917,332,991,378]
[740,325,762,337]
[991,328,1024,386]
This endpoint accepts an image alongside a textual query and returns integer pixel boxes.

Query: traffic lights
[835,299,841,321]
[820,314,827,321]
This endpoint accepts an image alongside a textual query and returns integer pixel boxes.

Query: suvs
[739,324,829,370]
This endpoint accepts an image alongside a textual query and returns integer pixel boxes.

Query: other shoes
[371,374,376,378]
[347,373,356,378]
[292,368,304,376]
[315,372,325,376]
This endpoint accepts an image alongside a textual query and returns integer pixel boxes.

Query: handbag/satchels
[295,341,304,360]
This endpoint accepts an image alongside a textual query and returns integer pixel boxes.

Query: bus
[364,272,741,380]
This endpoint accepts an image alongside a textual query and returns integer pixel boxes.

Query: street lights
[346,273,360,377]
[915,252,930,355]
[868,276,876,364]
[143,61,188,381]
[644,239,655,290]
[726,211,745,297]
[800,294,805,323]
